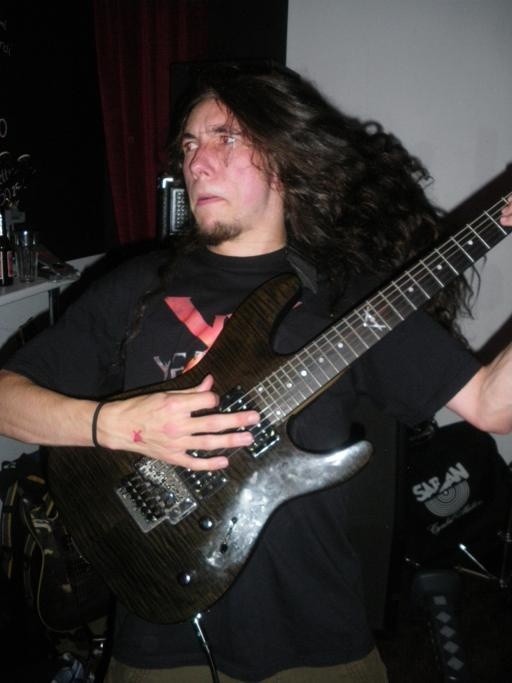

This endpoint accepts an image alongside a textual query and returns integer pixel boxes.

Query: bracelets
[93,401,107,450]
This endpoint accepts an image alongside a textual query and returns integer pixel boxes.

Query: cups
[13,229,40,282]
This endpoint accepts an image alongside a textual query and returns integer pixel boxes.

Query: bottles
[0,213,15,287]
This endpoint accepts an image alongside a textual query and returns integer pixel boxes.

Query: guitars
[40,192,512,626]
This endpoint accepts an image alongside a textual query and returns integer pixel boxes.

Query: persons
[0,53,510,682]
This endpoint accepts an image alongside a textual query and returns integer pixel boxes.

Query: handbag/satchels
[3,452,110,632]
